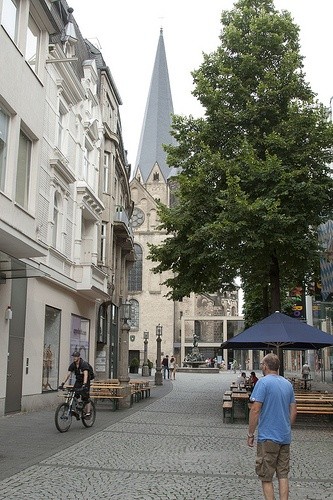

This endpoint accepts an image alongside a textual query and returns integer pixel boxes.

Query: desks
[94,379,119,384]
[301,379,311,390]
[65,384,124,394]
[231,392,249,424]
[233,388,247,392]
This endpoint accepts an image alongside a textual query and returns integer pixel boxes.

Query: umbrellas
[219,310,333,376]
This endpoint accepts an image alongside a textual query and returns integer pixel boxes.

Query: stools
[247,390,252,395]
[225,391,233,395]
[230,384,238,391]
[222,394,231,401]
[222,401,232,423]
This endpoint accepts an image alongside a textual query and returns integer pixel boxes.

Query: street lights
[155,323,164,384]
[142,329,149,377]
[118,299,133,410]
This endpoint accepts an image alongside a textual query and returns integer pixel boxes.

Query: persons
[168,358,176,381]
[163,355,171,379]
[59,351,91,417]
[204,357,226,370]
[238,372,247,385]
[302,362,310,379]
[248,372,259,386]
[231,359,238,373]
[238,363,242,372]
[248,353,297,499]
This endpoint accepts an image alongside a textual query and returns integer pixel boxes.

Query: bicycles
[53,386,97,433]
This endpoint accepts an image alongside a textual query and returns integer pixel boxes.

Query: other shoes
[84,412,91,417]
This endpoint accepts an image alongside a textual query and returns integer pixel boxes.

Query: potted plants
[130,357,140,373]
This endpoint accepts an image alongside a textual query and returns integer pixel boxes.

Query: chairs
[129,379,151,406]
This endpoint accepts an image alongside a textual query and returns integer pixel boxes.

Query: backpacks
[82,361,94,380]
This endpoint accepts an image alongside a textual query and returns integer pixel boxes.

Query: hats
[72,351,80,357]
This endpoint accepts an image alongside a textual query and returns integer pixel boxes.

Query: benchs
[65,395,123,410]
[293,388,333,414]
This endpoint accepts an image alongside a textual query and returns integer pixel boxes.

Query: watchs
[248,432,254,438]
[82,383,86,385]
